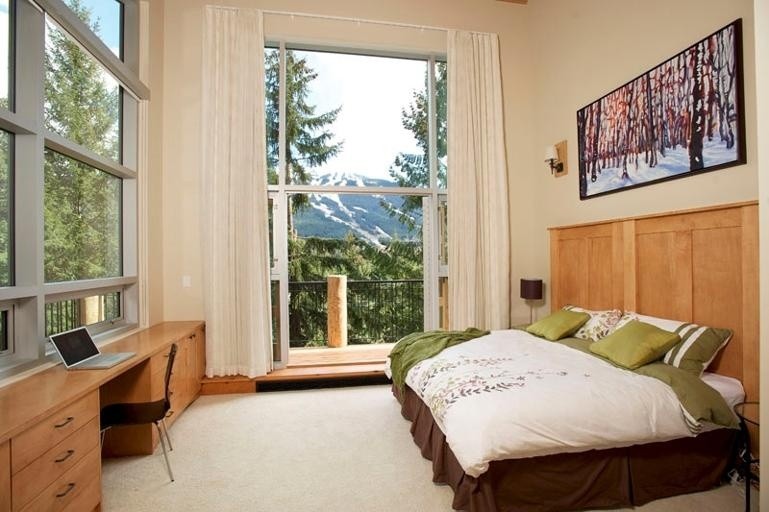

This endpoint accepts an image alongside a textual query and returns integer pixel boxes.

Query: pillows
[525,303,733,380]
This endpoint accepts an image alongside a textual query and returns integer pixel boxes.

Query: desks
[0,320,207,511]
[732,401,760,511]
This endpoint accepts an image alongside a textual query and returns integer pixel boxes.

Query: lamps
[520,277,542,324]
[543,139,568,178]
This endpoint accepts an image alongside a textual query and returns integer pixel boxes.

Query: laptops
[48,326,135,369]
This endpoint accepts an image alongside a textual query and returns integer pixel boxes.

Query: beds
[389,198,758,511]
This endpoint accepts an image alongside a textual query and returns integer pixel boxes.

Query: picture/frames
[576,18,747,201]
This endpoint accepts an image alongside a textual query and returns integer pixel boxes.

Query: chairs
[99,342,178,482]
